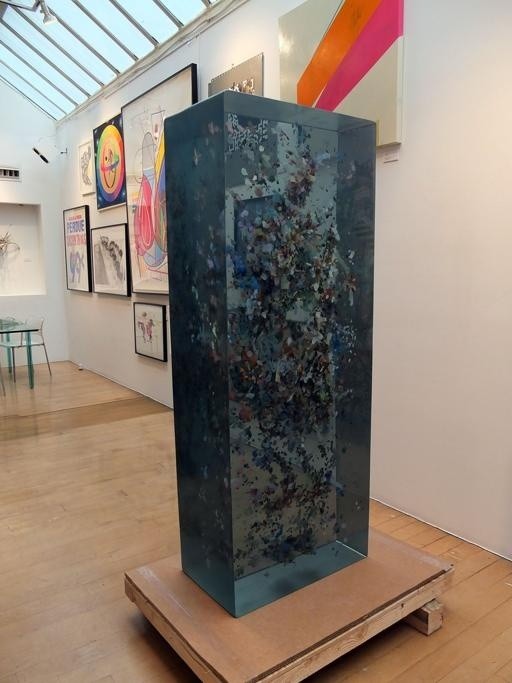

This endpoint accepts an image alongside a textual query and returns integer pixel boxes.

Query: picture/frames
[133,300,167,363]
[61,62,197,297]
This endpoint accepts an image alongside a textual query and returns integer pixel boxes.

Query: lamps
[34,0,57,24]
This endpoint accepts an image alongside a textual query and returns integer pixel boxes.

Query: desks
[0,318,39,392]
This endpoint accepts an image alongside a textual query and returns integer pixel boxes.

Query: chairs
[0,314,52,383]
